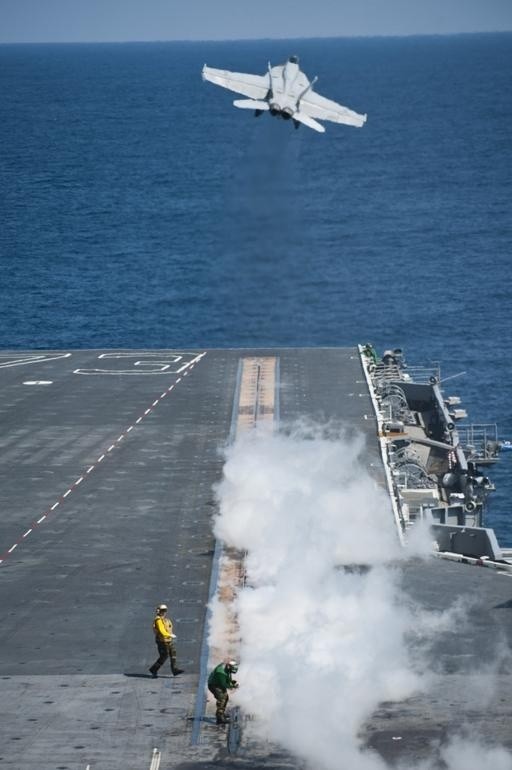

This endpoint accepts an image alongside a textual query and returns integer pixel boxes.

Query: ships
[1,341,512,770]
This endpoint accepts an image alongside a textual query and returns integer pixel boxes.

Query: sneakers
[173,670,184,676]
[216,712,230,724]
[149,669,158,678]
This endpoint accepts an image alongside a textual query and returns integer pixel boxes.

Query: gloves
[229,680,238,688]
[169,634,176,639]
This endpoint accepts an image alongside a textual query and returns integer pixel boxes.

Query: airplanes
[201,55,368,135]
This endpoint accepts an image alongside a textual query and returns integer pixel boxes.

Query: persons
[148,604,184,677]
[207,656,240,725]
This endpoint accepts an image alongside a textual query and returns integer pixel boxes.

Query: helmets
[226,661,238,674]
[156,605,167,615]
